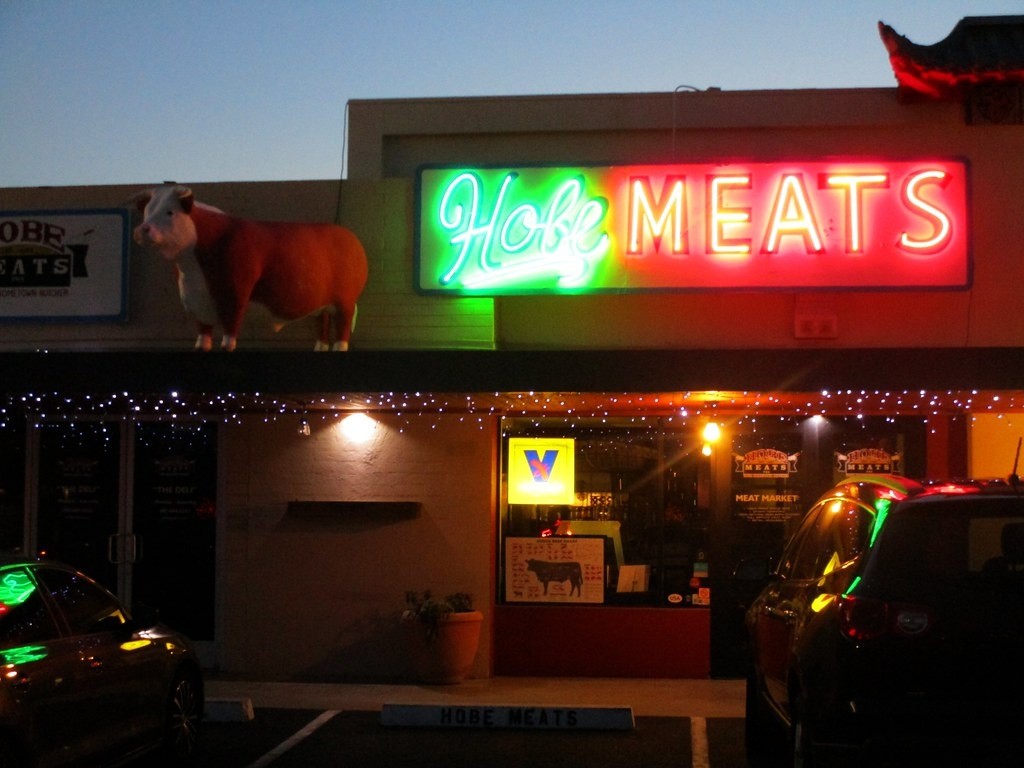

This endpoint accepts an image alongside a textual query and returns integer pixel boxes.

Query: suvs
[744,470,1024,768]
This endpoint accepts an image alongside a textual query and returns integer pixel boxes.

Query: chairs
[977,521,1024,591]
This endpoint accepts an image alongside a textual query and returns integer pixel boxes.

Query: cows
[525,558,583,597]
[133,186,368,351]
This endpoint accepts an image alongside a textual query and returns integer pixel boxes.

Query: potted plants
[406,589,483,684]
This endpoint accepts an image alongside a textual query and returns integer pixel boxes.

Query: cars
[0,563,209,768]
